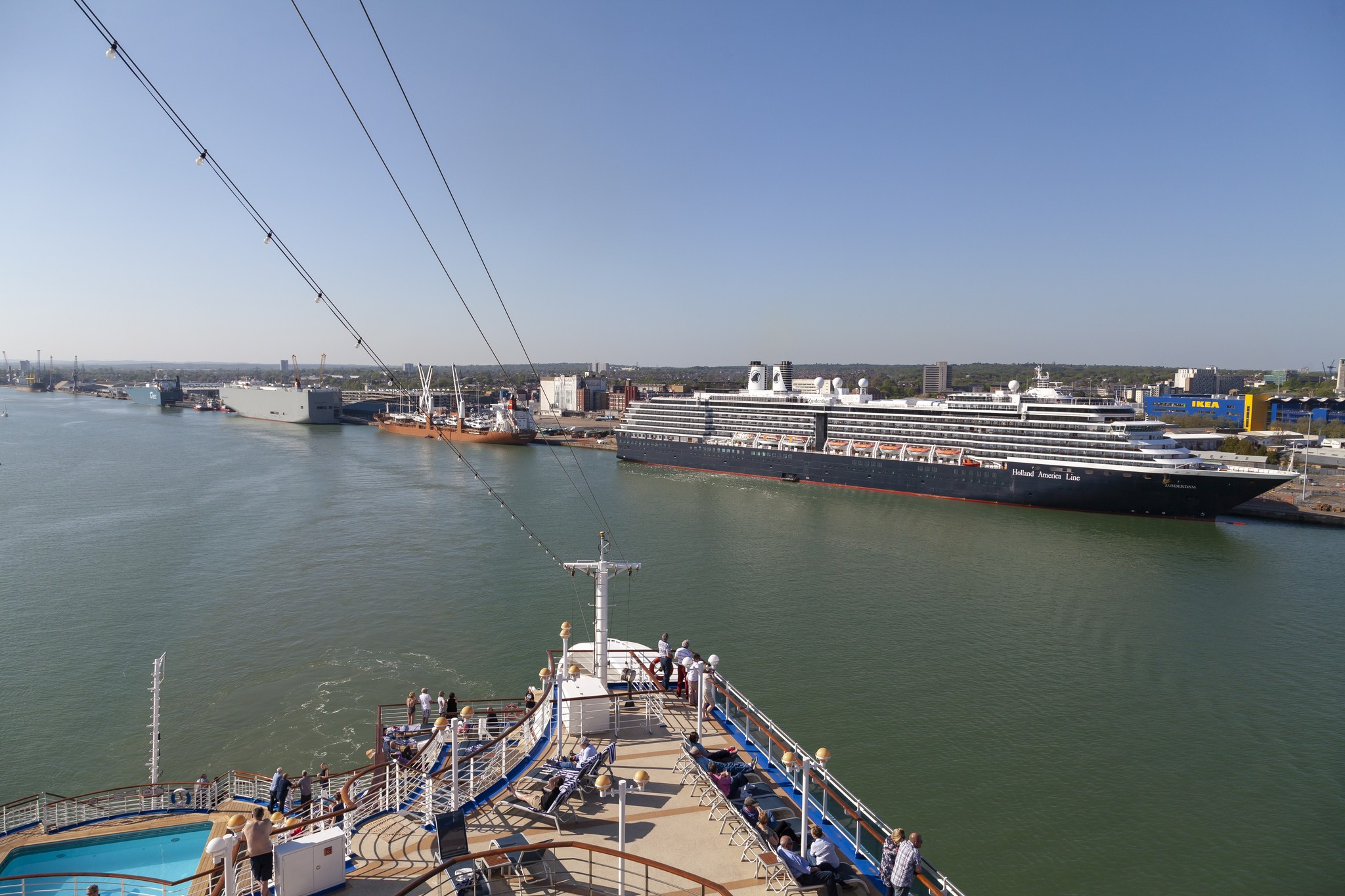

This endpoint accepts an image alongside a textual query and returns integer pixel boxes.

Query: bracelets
[530,699,531,701]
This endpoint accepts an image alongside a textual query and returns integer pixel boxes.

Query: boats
[0,526,968,896]
[2,411,8,417]
[194,399,234,413]
[371,400,537,445]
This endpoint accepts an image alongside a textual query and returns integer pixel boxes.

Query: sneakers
[740,791,752,798]
[729,748,739,757]
[724,746,735,752]
[751,758,756,772]
[750,756,758,771]
[744,783,756,790]
[666,687,671,691]
[546,759,554,765]
[555,751,561,760]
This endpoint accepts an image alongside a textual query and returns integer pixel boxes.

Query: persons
[317,763,329,802]
[879,826,923,896]
[418,688,434,724]
[658,633,717,721]
[689,731,848,885]
[195,774,210,809]
[437,691,447,718]
[524,686,536,723]
[296,770,314,818]
[240,806,275,896]
[506,775,566,810]
[266,767,283,814]
[325,793,366,831]
[209,777,223,809]
[485,706,498,728]
[277,773,296,814]
[406,692,418,725]
[446,692,458,721]
[86,884,101,896]
[456,719,469,736]
[389,740,427,766]
[546,736,598,771]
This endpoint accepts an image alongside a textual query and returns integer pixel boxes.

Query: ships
[615,361,1301,519]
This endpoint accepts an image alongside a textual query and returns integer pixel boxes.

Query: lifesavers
[171,788,191,808]
[649,657,674,681]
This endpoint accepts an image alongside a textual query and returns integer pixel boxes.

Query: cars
[532,416,612,445]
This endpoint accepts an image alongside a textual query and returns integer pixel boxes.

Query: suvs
[1308,478,1313,483]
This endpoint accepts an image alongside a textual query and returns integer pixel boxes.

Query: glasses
[86,890,97,896]
[287,775,289,776]
[689,644,690,645]
[281,769,283,772]
[787,841,794,845]
[487,708,492,711]
[696,657,701,660]
[901,834,906,839]
[914,840,923,844]
[580,743,585,747]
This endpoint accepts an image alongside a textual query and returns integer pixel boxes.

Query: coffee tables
[541,783,552,795]
[481,853,512,886]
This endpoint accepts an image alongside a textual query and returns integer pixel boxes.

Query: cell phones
[541,788,546,795]
[292,779,295,784]
[570,759,574,762]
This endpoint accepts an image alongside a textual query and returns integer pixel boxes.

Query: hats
[438,690,445,696]
[421,688,429,692]
[744,796,759,807]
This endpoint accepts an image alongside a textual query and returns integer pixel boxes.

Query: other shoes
[409,756,419,760]
[842,884,858,893]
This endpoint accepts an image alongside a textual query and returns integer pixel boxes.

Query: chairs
[671,728,871,896]
[514,752,602,812]
[488,832,554,896]
[433,808,494,896]
[383,721,510,823]
[702,439,1008,469]
[541,741,618,794]
[491,778,581,835]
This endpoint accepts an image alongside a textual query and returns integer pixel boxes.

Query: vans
[1312,480,1318,486]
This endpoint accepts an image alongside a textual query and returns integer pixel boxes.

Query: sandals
[694,701,698,705]
[689,702,697,706]
